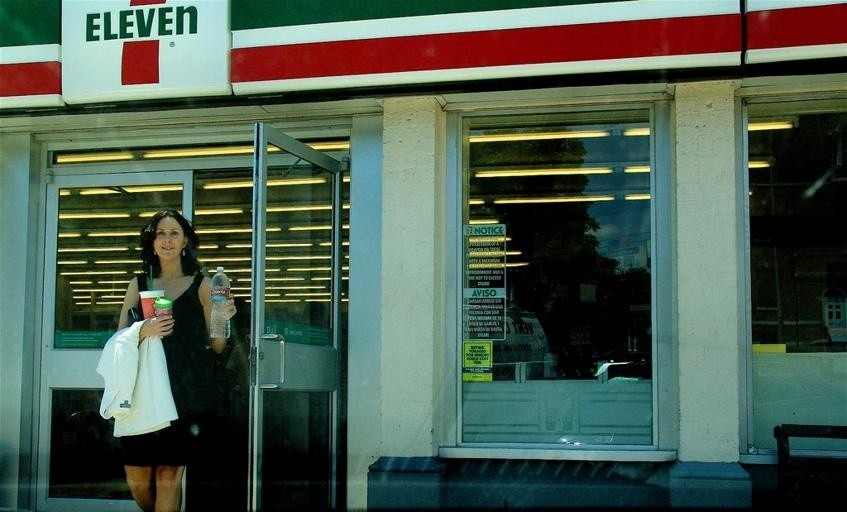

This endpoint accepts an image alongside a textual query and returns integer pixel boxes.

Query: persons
[94,208,238,511]
[302,302,326,325]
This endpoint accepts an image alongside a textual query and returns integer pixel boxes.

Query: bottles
[210,266,232,338]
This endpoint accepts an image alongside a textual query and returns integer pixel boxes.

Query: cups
[153,296,173,321]
[136,288,166,320]
